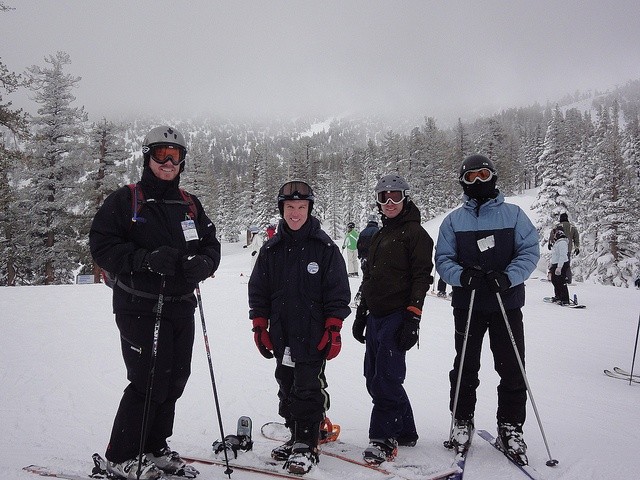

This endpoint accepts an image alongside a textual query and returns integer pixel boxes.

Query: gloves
[486,272,511,293]
[134,242,179,276]
[252,316,273,359]
[352,312,366,344]
[318,317,341,360]
[176,254,215,280]
[396,303,421,350]
[460,267,483,290]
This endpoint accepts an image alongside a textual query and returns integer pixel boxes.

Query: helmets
[276,180,315,220]
[458,154,499,199]
[141,126,188,175]
[560,213,569,223]
[552,224,566,240]
[374,174,412,217]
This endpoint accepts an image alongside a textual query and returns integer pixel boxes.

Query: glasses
[376,191,406,205]
[459,168,497,185]
[279,181,314,198]
[148,143,187,165]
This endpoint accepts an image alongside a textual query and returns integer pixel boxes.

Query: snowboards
[261,421,460,479]
[164,440,321,480]
[543,297,586,308]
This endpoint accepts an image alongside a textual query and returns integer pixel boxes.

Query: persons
[90,127,221,480]
[549,228,572,304]
[248,181,351,474]
[352,174,434,464]
[435,155,540,454]
[342,221,359,277]
[548,212,579,304]
[357,221,379,271]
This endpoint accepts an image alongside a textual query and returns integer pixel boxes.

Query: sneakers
[497,421,528,467]
[286,439,318,474]
[271,434,293,461]
[213,435,239,460]
[396,425,418,448]
[346,222,356,229]
[436,291,446,297]
[363,438,399,465]
[558,301,570,306]
[107,452,164,480]
[136,447,200,479]
[449,411,476,453]
[354,291,362,306]
[239,435,254,451]
[353,273,358,276]
[347,274,354,277]
[552,296,561,301]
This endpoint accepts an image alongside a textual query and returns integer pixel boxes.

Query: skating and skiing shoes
[449,292,453,297]
